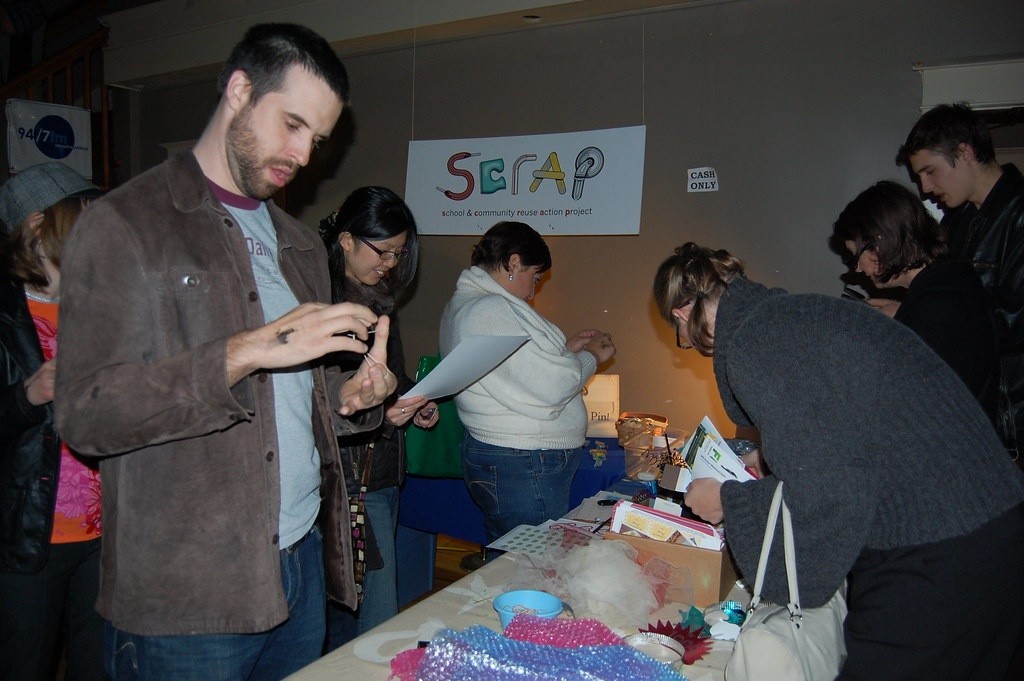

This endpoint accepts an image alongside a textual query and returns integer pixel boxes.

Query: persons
[833,100,1024,472]
[53,20,398,681]
[0,159,109,681]
[321,185,440,637]
[439,219,617,561]
[653,240,1024,681]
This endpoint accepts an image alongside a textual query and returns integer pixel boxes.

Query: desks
[394,437,627,609]
[280,479,850,681]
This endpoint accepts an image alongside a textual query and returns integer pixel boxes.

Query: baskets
[615,412,668,450]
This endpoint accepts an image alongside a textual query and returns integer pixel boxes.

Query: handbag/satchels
[349,497,384,603]
[724,480,848,681]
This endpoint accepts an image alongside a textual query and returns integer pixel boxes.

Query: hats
[0,161,99,237]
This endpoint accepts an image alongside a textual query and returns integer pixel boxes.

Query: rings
[402,407,405,412]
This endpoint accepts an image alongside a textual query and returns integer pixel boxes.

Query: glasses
[844,241,873,271]
[359,238,409,260]
[676,299,693,349]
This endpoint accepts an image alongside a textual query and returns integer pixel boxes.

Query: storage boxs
[622,426,691,480]
[602,531,738,609]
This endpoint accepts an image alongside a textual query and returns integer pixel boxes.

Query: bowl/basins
[493,589,563,632]
[622,633,684,672]
